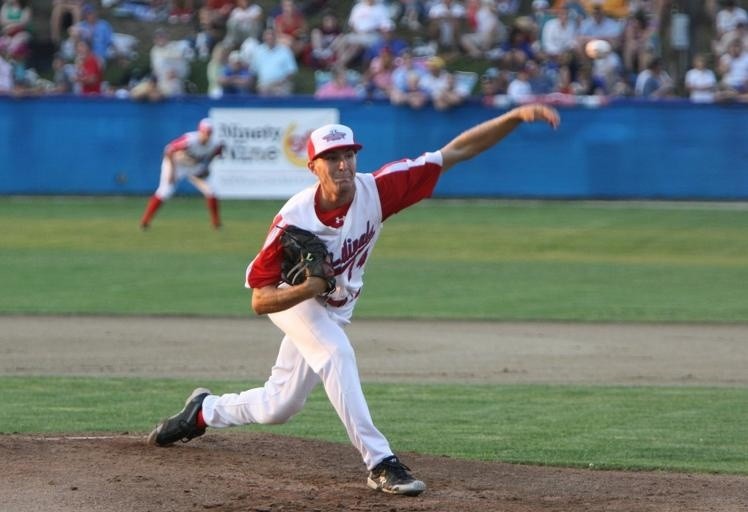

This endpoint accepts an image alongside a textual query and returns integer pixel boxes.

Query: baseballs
[585,39,611,58]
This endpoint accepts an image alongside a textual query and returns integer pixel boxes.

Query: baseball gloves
[280,224,336,296]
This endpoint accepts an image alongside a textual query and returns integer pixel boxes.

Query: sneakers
[146,385,211,448]
[363,455,427,497]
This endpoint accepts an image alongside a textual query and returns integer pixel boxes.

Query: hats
[306,124,364,163]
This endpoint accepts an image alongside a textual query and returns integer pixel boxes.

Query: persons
[137,116,229,231]
[142,102,563,495]
[0,0,748,111]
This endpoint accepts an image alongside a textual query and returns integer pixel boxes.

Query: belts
[317,287,363,308]
